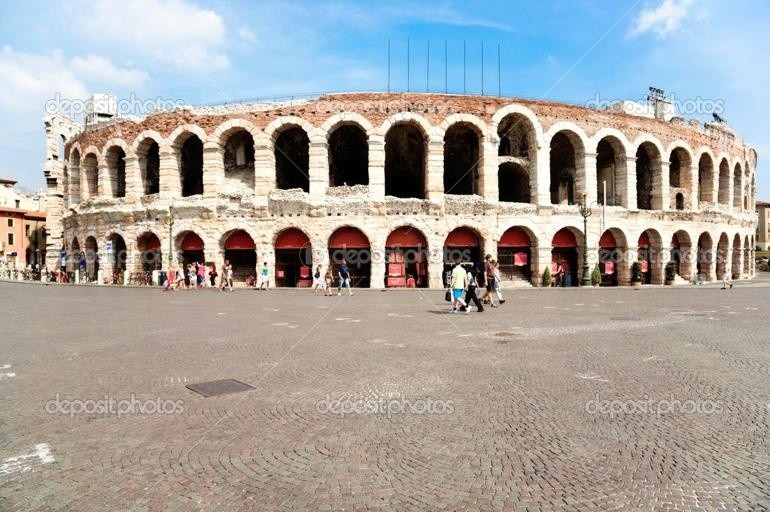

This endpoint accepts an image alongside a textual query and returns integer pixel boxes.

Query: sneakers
[465,304,472,314]
[484,301,490,304]
[448,308,457,314]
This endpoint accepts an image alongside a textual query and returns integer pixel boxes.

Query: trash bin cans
[564,272,571,287]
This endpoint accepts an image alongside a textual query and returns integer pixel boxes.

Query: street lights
[579,194,593,285]
[166,214,175,267]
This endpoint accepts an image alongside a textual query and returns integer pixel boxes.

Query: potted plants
[544,266,553,286]
[664,260,677,286]
[630,262,642,288]
[591,262,602,286]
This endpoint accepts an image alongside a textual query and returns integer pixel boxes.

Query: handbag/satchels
[446,289,451,301]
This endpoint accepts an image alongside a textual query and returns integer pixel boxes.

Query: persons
[324,265,335,296]
[0,247,270,298]
[552,262,566,287]
[407,275,416,288]
[336,259,356,297]
[313,265,326,293]
[447,254,505,315]
[721,261,735,290]
